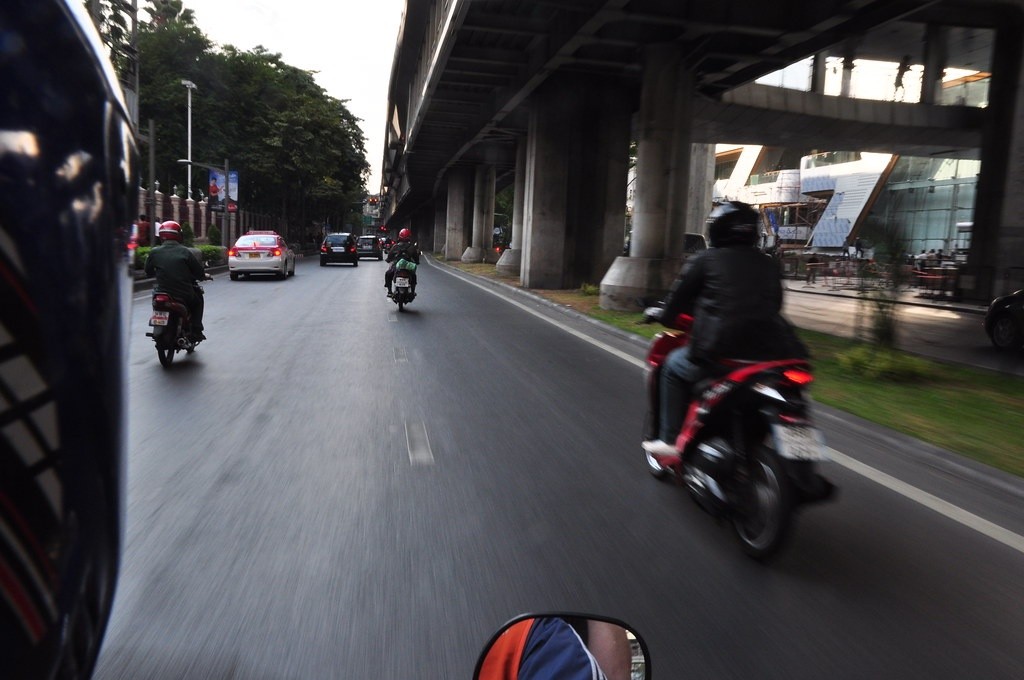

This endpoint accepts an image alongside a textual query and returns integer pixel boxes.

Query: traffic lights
[369,195,378,204]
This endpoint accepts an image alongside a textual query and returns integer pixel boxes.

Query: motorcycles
[144,260,217,367]
[385,246,419,311]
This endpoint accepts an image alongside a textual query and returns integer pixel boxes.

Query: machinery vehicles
[757,203,809,260]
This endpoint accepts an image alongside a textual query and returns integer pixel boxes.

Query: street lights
[178,159,229,251]
[180,79,198,199]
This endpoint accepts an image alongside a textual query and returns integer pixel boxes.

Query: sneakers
[641,439,683,457]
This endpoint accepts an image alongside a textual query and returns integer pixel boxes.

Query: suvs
[683,233,708,258]
[357,236,383,261]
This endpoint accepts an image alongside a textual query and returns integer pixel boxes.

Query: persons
[307,232,324,250]
[133,215,162,248]
[384,228,419,297]
[479,617,632,680]
[642,201,808,455]
[209,177,224,200]
[854,237,864,258]
[145,221,210,341]
[901,248,942,269]
[806,253,819,284]
[842,236,850,259]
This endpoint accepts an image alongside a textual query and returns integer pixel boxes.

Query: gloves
[645,307,664,322]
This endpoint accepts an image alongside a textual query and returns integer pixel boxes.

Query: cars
[228,231,296,278]
[320,233,359,266]
[494,243,510,253]
[982,289,1024,349]
[380,238,392,248]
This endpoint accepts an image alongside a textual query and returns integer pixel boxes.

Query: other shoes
[193,332,206,340]
[386,293,393,297]
[412,292,417,296]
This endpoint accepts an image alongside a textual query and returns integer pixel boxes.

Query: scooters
[386,244,390,252]
[631,303,840,560]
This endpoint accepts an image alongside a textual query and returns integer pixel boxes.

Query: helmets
[706,202,759,247]
[399,228,411,239]
[159,221,184,242]
